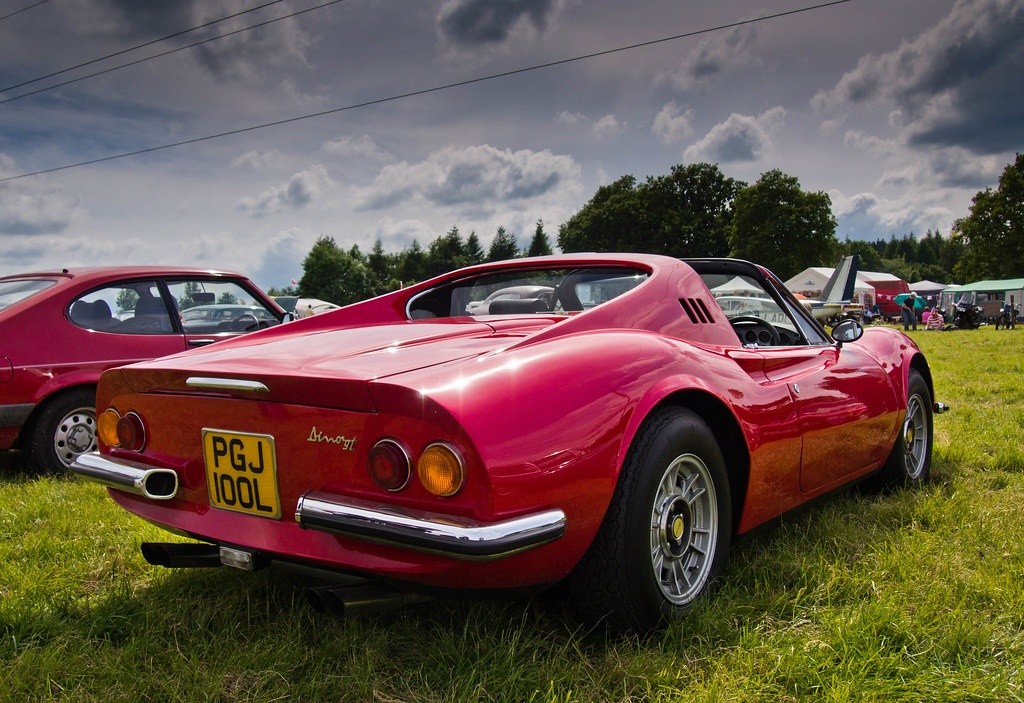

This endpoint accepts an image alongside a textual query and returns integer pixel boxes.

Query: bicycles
[995,301,1021,330]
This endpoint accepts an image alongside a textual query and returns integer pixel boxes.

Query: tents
[783,267,876,305]
[710,276,765,298]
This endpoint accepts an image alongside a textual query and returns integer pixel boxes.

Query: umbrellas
[893,293,927,308]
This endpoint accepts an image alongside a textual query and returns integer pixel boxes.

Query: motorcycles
[954,292,984,330]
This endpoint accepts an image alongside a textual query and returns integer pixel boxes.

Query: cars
[468,286,562,316]
[180,305,282,334]
[0,268,306,478]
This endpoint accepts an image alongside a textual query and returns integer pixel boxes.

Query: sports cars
[67,252,951,643]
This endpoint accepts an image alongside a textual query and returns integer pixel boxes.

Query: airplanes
[719,254,865,332]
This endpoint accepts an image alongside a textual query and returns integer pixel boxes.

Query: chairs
[489,298,548,314]
[71,299,112,328]
[135,295,180,333]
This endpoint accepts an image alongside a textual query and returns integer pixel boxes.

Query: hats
[911,292,916,297]
[924,306,929,310]
[937,305,941,308]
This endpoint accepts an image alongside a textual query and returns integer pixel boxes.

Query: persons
[304,305,314,318]
[902,292,917,330]
[922,307,945,331]
